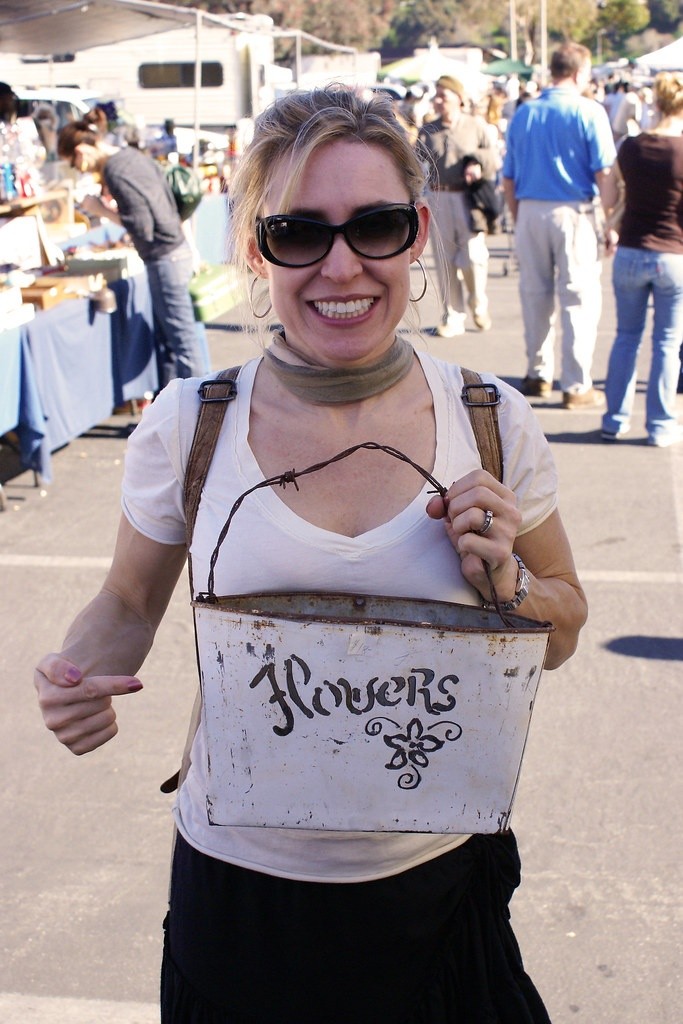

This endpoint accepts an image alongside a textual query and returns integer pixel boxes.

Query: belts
[431,183,466,193]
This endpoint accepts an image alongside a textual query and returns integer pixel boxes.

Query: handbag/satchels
[167,167,201,221]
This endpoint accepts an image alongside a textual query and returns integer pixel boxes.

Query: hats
[436,75,465,106]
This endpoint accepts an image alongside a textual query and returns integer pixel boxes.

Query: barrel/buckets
[189,440,557,838]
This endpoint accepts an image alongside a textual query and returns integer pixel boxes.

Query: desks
[181,192,236,271]
[1,272,159,487]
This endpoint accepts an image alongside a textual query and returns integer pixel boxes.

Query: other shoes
[648,425,683,447]
[564,388,606,410]
[468,300,492,331]
[599,427,621,440]
[437,319,466,339]
[522,374,554,397]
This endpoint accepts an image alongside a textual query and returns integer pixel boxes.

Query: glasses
[69,154,77,170]
[256,200,419,269]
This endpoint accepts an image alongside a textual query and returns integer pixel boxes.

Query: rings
[480,509,493,532]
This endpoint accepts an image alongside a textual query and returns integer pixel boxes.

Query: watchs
[483,551,529,612]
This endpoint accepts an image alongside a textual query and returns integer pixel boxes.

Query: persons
[600,71,683,445]
[58,108,212,392]
[0,72,642,233]
[32,80,589,1023]
[503,43,616,408]
[414,74,504,339]
